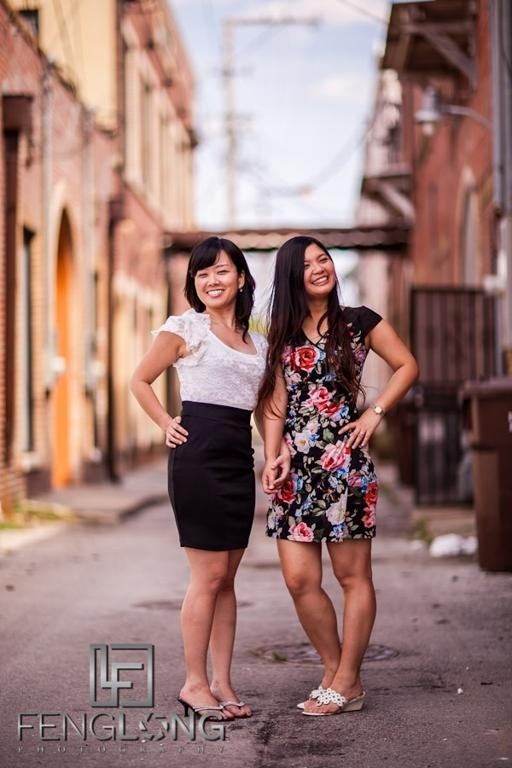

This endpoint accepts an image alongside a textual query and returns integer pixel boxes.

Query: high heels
[297,687,366,716]
[178,695,250,721]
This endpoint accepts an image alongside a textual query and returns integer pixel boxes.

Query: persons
[259,235,421,716]
[127,233,291,725]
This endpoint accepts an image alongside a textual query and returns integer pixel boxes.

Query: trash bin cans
[458,380,512,571]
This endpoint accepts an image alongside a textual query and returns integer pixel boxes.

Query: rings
[360,430,365,436]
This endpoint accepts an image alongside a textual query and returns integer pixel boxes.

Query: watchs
[369,402,386,418]
